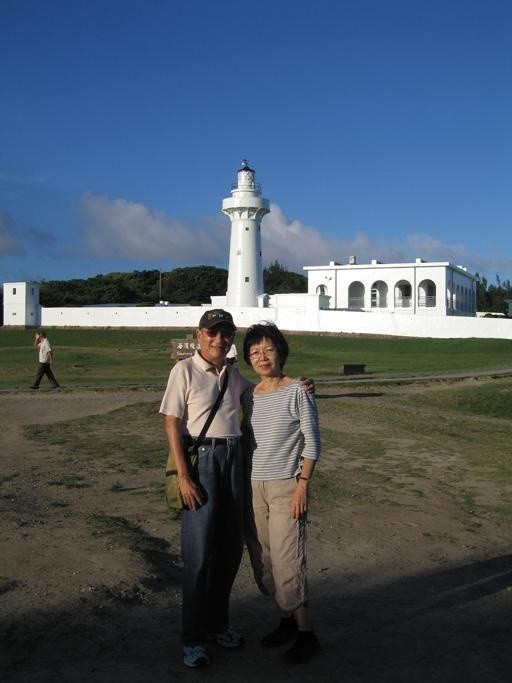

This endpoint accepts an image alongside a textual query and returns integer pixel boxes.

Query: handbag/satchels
[164,445,198,509]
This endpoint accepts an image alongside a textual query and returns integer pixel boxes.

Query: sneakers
[282,632,321,663]
[206,626,246,647]
[183,644,210,667]
[261,616,298,645]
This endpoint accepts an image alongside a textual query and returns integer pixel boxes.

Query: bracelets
[297,476,309,481]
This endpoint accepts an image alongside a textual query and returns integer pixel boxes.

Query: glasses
[201,327,233,338]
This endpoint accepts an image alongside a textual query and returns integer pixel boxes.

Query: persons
[29,330,60,390]
[226,343,238,367]
[239,320,322,662]
[157,307,316,669]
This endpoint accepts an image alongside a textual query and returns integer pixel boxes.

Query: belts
[184,435,245,446]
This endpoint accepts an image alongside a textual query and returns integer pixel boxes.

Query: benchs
[337,364,367,375]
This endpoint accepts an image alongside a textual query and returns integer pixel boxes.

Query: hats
[198,308,237,330]
